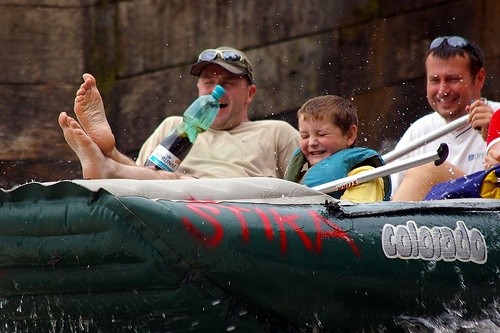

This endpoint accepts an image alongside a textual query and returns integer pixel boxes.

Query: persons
[57,47,303,180]
[387,36,500,202]
[286,96,392,201]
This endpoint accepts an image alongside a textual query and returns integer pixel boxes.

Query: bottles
[143,84,226,173]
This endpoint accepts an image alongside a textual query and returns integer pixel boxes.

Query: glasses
[429,36,469,49]
[198,49,242,62]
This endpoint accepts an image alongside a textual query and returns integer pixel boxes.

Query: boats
[0,177,500,333]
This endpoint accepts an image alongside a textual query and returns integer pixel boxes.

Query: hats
[190,47,255,84]
